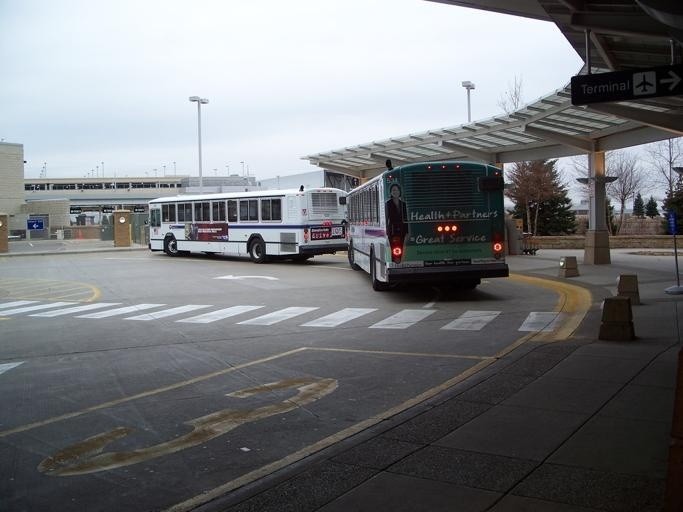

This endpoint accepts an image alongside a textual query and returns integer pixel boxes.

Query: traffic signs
[25,219,43,231]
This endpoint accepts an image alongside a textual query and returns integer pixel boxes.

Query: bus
[338,157,512,296]
[142,183,350,265]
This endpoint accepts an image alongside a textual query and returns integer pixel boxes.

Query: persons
[384,182,408,263]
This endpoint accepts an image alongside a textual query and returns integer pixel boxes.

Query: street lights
[188,95,211,195]
[212,160,245,176]
[459,79,476,124]
[143,161,177,177]
[38,160,47,178]
[81,159,105,179]
